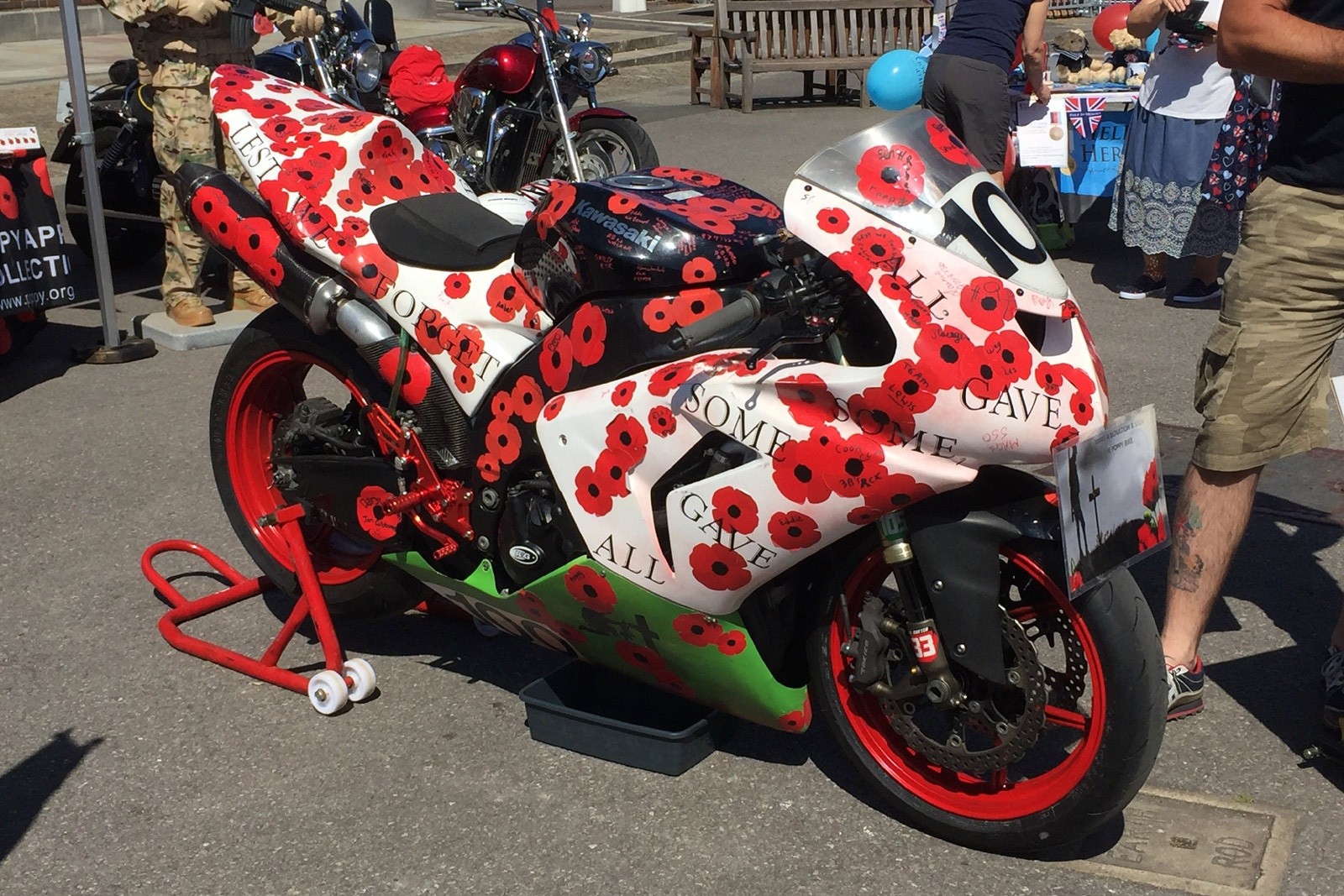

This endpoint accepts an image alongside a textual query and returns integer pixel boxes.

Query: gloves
[167,0,231,25]
[290,6,324,37]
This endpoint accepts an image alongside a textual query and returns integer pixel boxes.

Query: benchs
[686,0,958,113]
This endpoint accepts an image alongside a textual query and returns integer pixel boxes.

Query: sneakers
[1317,646,1344,729]
[1119,276,1168,299]
[1173,278,1222,301]
[225,288,279,312]
[167,298,215,327]
[1079,650,1204,721]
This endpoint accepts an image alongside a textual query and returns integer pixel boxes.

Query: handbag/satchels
[1230,68,1272,107]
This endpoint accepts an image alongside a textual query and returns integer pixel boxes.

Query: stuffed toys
[1050,28,1110,84]
[1104,28,1155,87]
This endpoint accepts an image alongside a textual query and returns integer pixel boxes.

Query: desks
[1008,80,1141,223]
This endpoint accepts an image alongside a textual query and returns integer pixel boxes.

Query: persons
[97,0,324,328]
[1160,0,1344,768]
[1107,0,1281,303]
[923,0,1053,194]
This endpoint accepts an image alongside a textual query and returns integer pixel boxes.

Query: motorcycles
[357,0,659,199]
[51,1,383,276]
[172,64,1171,860]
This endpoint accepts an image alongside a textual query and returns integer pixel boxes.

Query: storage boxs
[518,657,722,777]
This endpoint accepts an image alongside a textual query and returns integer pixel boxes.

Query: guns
[224,0,347,49]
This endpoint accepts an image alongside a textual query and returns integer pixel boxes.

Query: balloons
[1093,3,1136,51]
[865,49,929,112]
